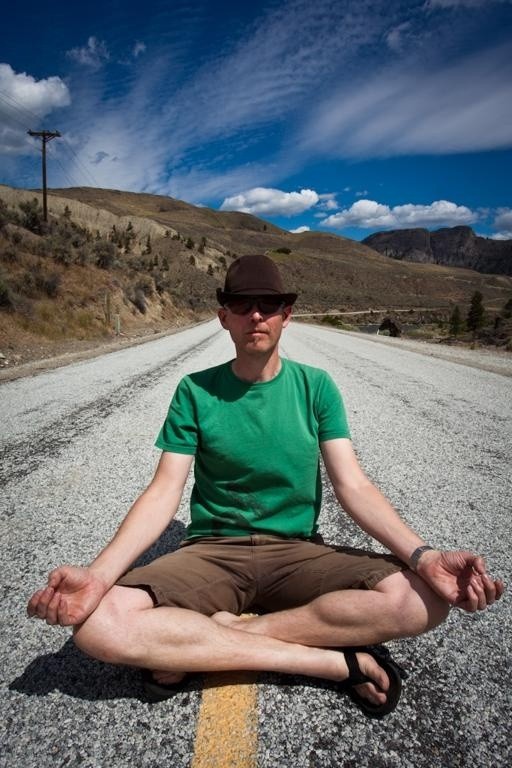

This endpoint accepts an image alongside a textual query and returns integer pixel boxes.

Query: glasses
[227,296,283,315]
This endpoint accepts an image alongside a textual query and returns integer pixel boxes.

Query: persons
[27,255,504,717]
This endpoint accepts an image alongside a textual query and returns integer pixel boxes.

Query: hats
[216,255,298,309]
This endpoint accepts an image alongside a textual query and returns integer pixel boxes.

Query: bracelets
[410,545,435,575]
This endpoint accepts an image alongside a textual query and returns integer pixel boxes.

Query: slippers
[147,670,189,689]
[339,648,402,715]
